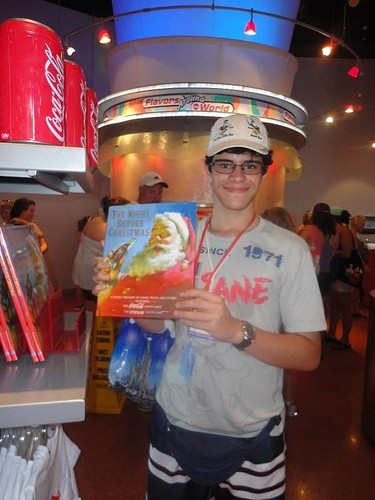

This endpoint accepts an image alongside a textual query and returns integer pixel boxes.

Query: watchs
[231,318,257,351]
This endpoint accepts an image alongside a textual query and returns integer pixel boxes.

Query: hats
[207,114,270,158]
[165,212,195,269]
[139,173,168,188]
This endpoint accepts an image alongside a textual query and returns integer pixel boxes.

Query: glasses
[207,159,264,175]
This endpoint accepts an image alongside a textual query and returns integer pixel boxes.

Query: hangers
[0,425,49,463]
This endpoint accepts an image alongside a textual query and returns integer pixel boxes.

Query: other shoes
[285,399,297,416]
[338,313,343,319]
[323,333,335,343]
[137,405,153,413]
[353,311,368,318]
[74,303,85,310]
[332,341,351,350]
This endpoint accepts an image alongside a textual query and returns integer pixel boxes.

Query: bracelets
[39,235,44,239]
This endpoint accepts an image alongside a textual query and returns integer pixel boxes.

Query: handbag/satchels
[107,318,174,404]
[153,402,281,484]
[340,261,364,287]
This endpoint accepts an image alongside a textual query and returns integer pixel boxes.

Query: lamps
[343,103,355,114]
[346,59,360,80]
[98,23,112,44]
[65,37,76,57]
[321,34,334,57]
[243,8,257,35]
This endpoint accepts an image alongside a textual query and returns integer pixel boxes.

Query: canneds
[0,18,99,171]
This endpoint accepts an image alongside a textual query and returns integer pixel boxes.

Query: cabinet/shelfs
[0,142,91,427]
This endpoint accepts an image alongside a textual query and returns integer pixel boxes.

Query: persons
[20,233,53,320]
[266,201,370,348]
[0,171,169,305]
[96,212,194,318]
[133,112,328,500]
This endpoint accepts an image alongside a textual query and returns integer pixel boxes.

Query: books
[0,200,198,365]
